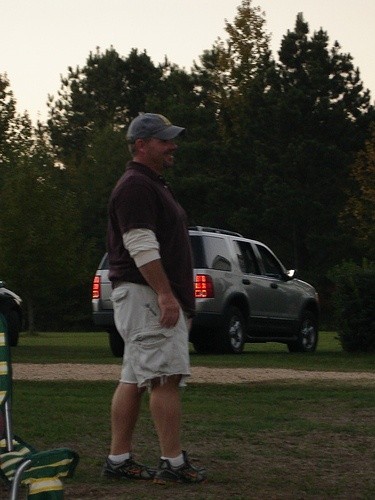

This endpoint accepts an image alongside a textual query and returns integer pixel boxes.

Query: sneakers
[103,449,209,485]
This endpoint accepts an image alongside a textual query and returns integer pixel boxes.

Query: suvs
[91,225,320,358]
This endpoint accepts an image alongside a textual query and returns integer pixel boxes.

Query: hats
[128,109,185,142]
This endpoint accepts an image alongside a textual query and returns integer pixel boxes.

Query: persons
[103,113,214,485]
